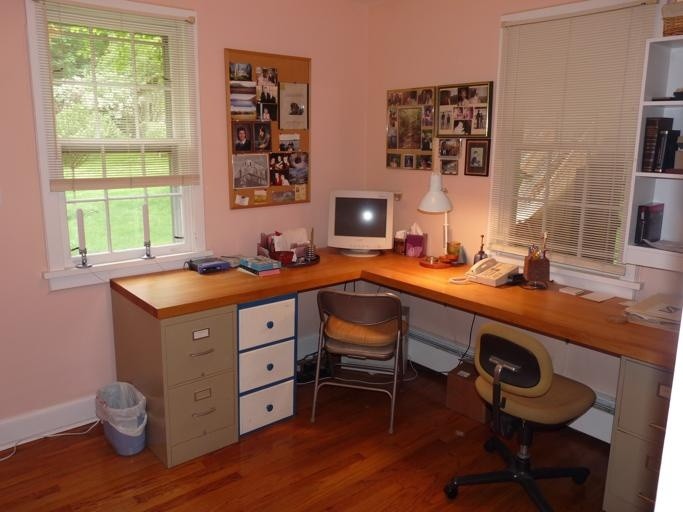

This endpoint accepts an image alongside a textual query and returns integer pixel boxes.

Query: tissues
[406,223,425,258]
[395,230,409,256]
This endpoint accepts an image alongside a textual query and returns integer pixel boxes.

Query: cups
[303,244,315,260]
[446,241,461,263]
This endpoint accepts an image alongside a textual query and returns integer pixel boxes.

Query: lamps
[419,170,459,270]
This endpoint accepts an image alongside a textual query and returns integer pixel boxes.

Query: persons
[267,91,275,103]
[256,128,270,151]
[235,128,251,152]
[267,73,274,83]
[440,142,449,156]
[471,151,482,167]
[262,108,270,120]
[440,86,487,138]
[269,144,306,186]
[261,87,269,102]
[387,89,433,170]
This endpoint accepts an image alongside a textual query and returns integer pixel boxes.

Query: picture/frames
[254,120,272,154]
[230,120,254,154]
[384,81,495,177]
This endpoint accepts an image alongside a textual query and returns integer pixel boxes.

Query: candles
[77,209,85,249]
[143,203,150,242]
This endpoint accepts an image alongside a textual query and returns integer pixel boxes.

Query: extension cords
[295,367,330,383]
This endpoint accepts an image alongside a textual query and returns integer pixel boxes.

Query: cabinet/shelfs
[619,34,683,275]
[600,352,674,512]
[111,289,238,468]
[239,291,298,437]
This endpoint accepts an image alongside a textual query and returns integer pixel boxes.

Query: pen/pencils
[527,232,549,261]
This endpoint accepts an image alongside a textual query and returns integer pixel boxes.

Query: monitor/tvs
[328,189,393,257]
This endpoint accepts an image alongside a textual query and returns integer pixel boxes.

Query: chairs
[306,290,409,435]
[442,321,595,511]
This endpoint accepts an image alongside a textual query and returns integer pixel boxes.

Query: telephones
[465,257,519,286]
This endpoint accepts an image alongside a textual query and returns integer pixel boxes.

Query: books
[641,117,674,172]
[236,256,281,278]
[652,129,680,172]
[623,290,682,333]
[634,201,664,247]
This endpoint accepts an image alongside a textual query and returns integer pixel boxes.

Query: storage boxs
[444,363,495,425]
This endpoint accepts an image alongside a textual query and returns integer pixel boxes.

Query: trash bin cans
[95,381,147,456]
